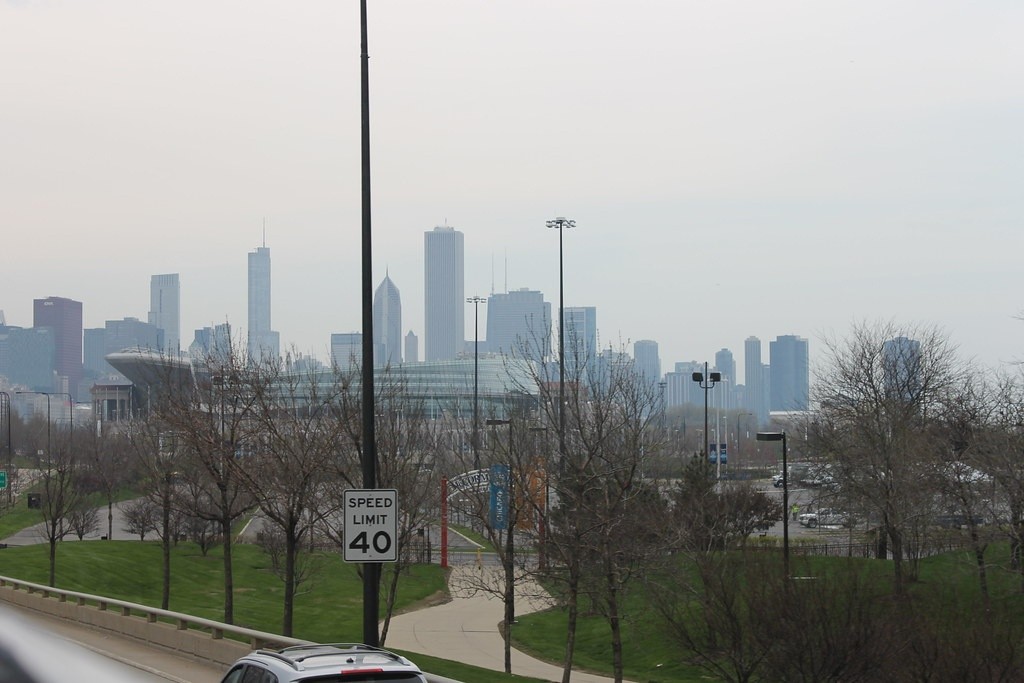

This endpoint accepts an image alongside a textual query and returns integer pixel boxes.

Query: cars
[933,509,985,531]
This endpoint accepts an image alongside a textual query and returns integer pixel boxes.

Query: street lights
[757,431,791,615]
[545,218,575,480]
[694,372,721,481]
[465,295,488,471]
[485,419,515,625]
[214,376,242,489]
[15,390,74,491]
[529,426,551,577]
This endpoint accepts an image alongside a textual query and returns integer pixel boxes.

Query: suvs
[799,508,856,529]
[221,643,428,683]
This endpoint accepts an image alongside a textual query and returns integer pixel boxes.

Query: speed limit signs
[343,489,398,564]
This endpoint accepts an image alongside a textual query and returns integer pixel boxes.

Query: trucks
[771,464,807,488]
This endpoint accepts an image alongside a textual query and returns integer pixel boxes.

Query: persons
[791,503,800,521]
